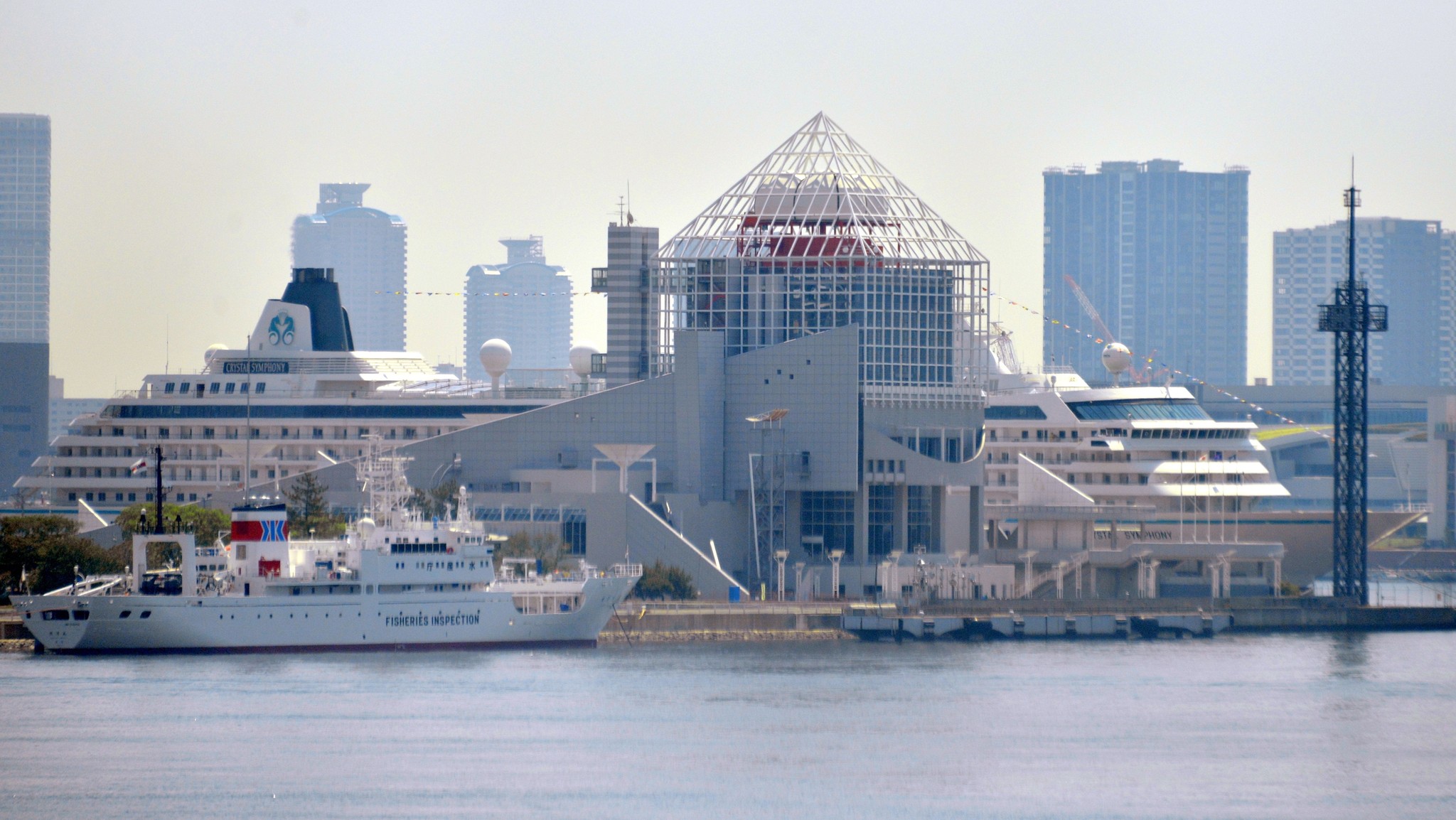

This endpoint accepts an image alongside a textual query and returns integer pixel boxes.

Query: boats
[2,263,599,537]
[982,342,1436,598]
[8,443,645,654]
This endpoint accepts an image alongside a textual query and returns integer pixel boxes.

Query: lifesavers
[330,572,335,580]
[447,547,453,554]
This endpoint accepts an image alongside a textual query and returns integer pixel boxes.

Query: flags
[1213,453,1223,460]
[1229,454,1236,463]
[375,290,607,298]
[1199,454,1208,462]
[129,457,147,475]
